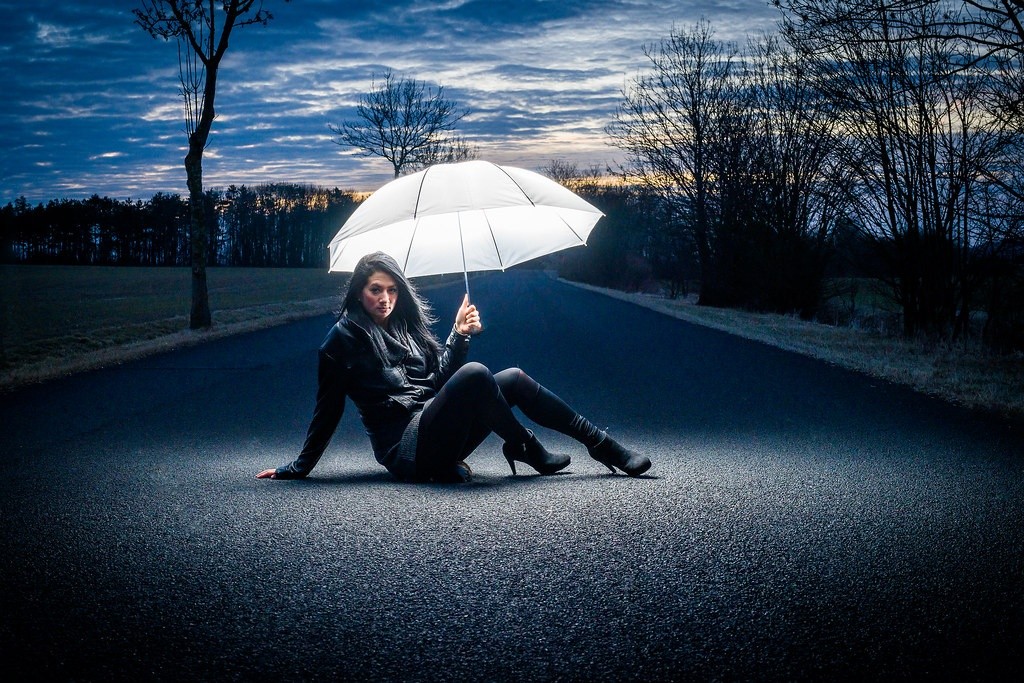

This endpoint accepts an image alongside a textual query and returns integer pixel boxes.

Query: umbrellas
[329,159,604,340]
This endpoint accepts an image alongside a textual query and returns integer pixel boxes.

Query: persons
[255,252,652,483]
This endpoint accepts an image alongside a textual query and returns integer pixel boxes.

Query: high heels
[502,428,571,476]
[587,430,653,478]
